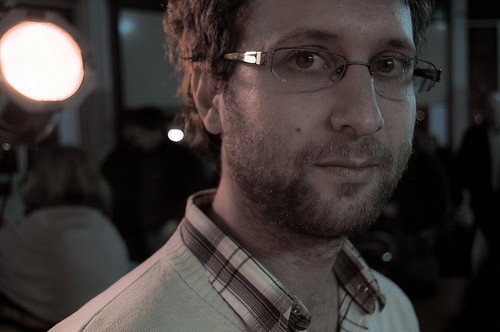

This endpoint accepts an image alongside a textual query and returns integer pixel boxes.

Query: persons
[0,106,500,332]
[49,0,429,332]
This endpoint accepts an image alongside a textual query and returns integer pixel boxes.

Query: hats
[0,208,129,322]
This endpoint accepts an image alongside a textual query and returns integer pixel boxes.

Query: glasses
[223,46,442,100]
